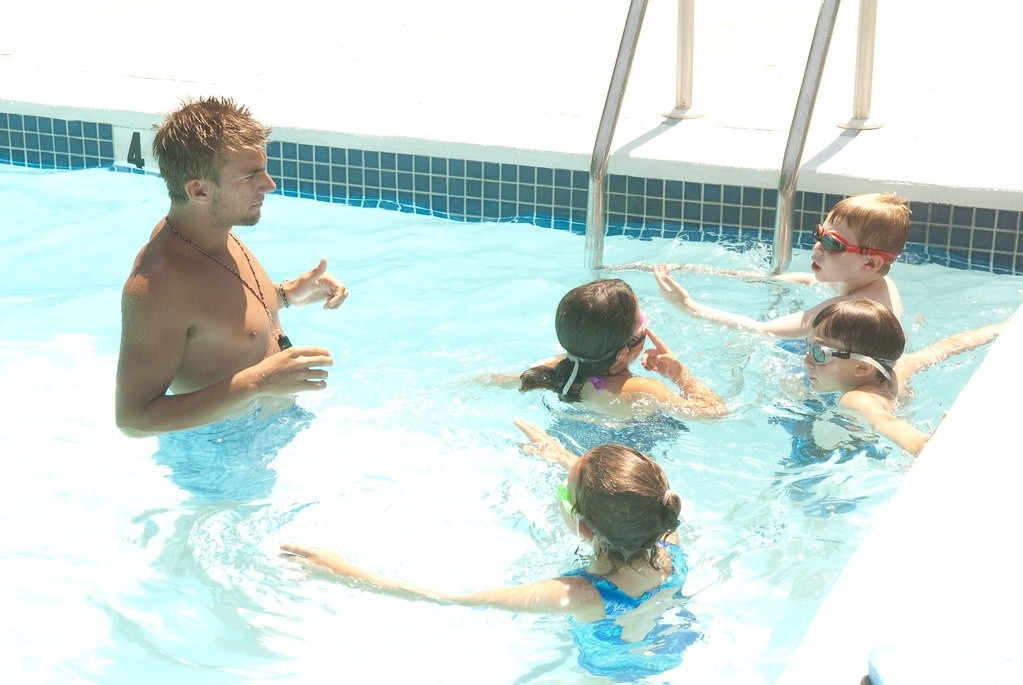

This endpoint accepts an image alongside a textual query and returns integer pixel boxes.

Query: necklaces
[164,215,293,352]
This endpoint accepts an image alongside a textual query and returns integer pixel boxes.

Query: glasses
[560,478,638,564]
[813,224,896,264]
[567,310,646,363]
[806,337,895,381]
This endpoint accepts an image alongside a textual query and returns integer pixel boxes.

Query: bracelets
[279,280,291,309]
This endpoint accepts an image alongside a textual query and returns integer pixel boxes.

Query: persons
[783,293,1010,519]
[113,98,348,512]
[280,417,702,682]
[475,278,728,462]
[654,192,912,405]
